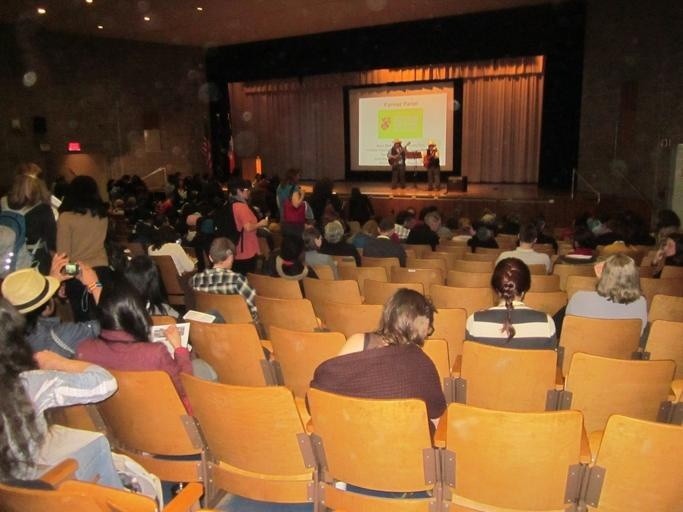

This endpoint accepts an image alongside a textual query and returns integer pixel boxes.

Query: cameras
[64,262,79,274]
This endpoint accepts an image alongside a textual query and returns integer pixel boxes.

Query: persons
[564,254,648,335]
[74,277,202,461]
[404,206,683,279]
[0,160,407,321]
[423,142,440,190]
[0,311,124,488]
[306,287,446,498]
[0,253,104,360]
[464,259,557,352]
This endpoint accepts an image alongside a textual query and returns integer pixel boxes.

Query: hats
[1,269,61,316]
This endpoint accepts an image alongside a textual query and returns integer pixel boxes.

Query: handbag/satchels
[284,198,306,224]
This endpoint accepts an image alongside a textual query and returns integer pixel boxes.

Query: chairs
[0,229,682,512]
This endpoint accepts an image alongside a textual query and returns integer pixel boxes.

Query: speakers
[34,116,46,133]
[211,108,232,182]
[447,176,467,192]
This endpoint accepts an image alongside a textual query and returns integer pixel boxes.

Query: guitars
[423,148,436,168]
[388,142,411,166]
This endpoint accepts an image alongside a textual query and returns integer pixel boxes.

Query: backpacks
[0,195,45,280]
[210,196,259,252]
